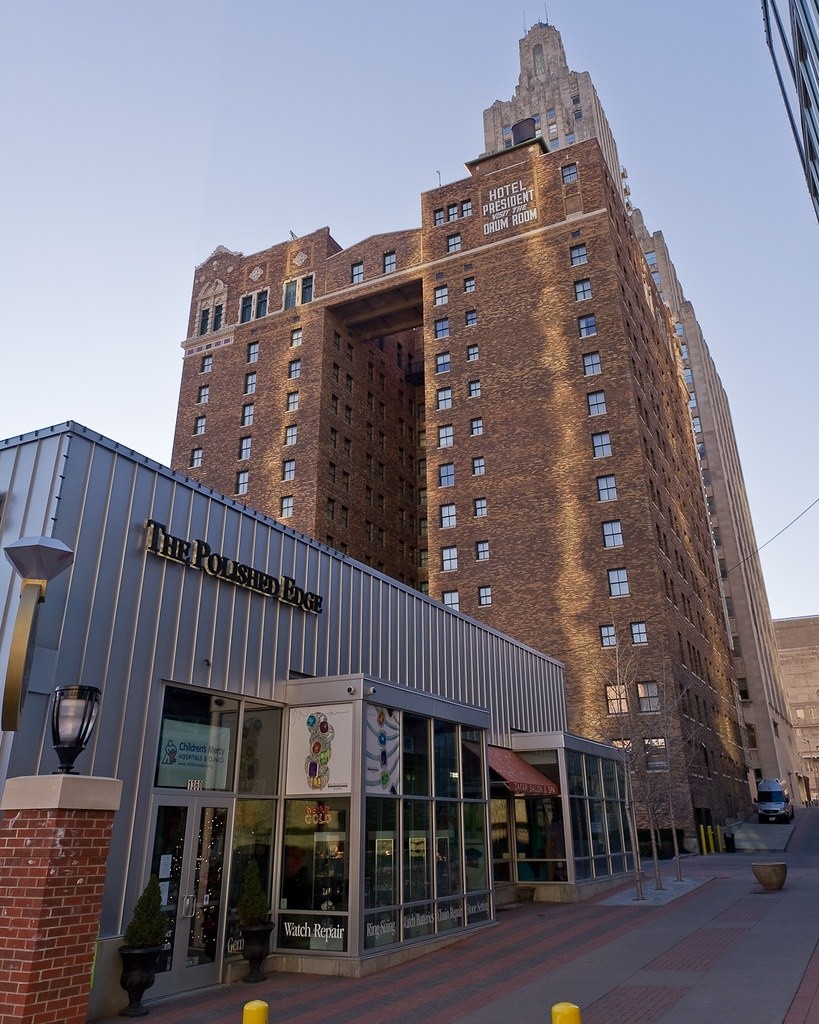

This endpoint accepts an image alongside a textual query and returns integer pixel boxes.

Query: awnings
[463,741,559,794]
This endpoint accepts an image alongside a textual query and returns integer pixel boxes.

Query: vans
[754,776,794,824]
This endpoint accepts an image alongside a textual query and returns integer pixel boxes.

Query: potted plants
[237,859,275,983]
[118,874,170,1017]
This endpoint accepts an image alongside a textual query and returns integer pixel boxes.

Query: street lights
[804,735,819,794]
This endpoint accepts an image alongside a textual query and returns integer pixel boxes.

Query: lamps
[52,685,102,774]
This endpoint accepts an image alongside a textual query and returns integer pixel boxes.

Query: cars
[207,844,349,910]
